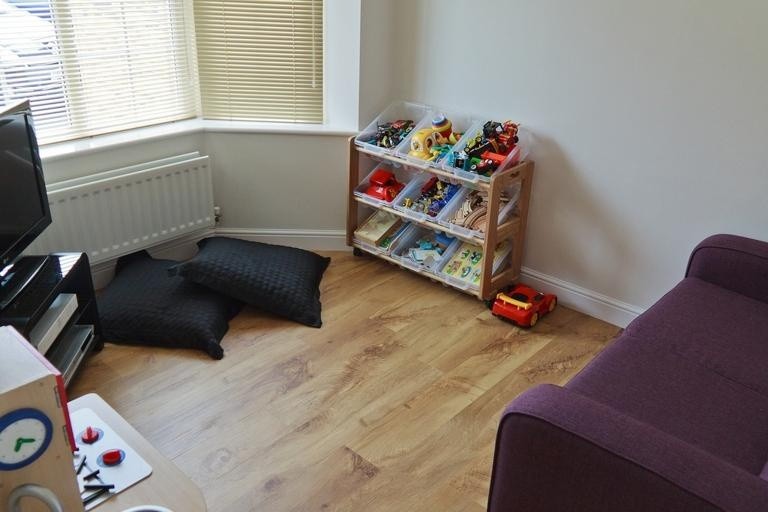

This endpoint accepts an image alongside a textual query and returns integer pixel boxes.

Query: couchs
[487,233,768,512]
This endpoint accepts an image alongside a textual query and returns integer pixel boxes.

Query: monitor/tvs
[0,99,55,312]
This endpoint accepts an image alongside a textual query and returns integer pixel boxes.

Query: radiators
[37,150,227,270]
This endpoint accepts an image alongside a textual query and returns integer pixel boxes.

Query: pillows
[85,237,331,363]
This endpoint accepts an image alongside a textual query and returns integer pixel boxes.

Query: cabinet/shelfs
[345,133,540,304]
[0,251,103,394]
